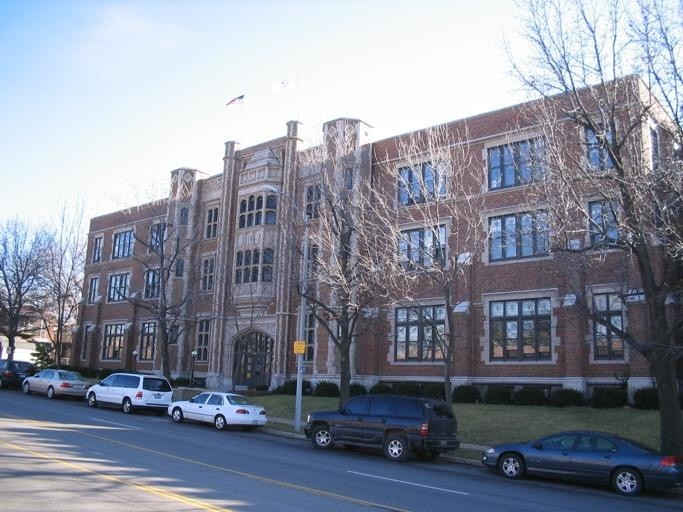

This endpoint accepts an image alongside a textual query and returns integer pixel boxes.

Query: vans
[85,373,173,414]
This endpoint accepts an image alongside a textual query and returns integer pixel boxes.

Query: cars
[480,430,680,497]
[167,391,266,431]
[21,369,92,399]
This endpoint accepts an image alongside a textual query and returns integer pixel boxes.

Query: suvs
[303,393,459,461]
[0,359,36,391]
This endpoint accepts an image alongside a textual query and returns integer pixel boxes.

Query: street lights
[189,350,196,388]
[257,183,307,433]
[131,350,137,372]
